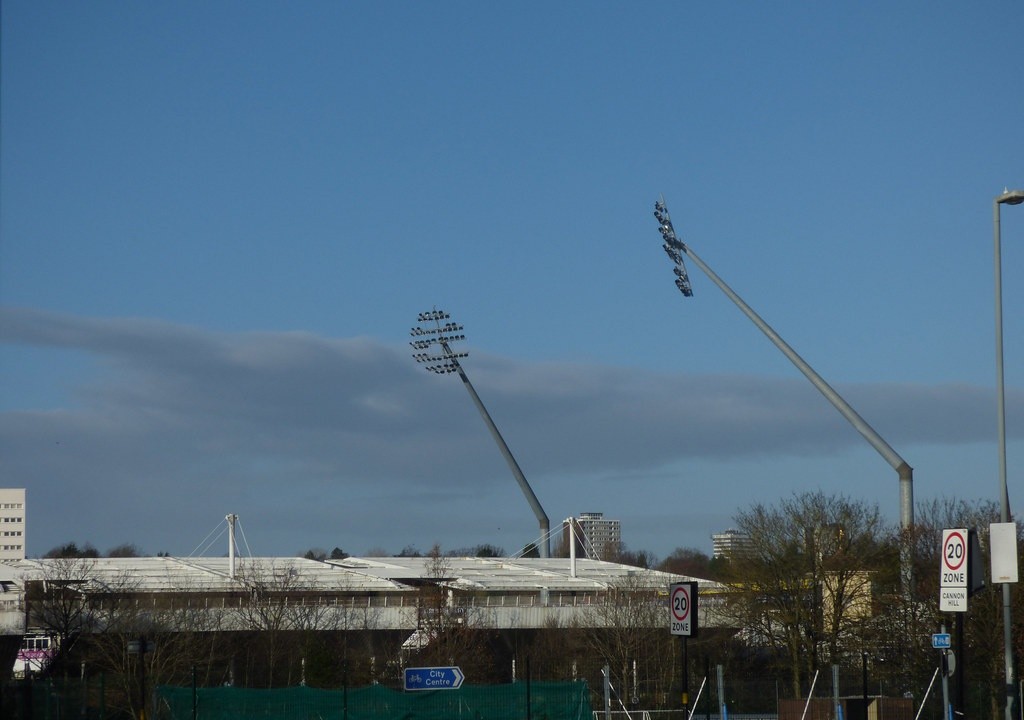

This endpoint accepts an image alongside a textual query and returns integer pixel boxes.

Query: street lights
[993,191,1024,720]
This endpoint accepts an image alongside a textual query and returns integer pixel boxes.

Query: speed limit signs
[940,529,968,587]
[670,584,691,635]
